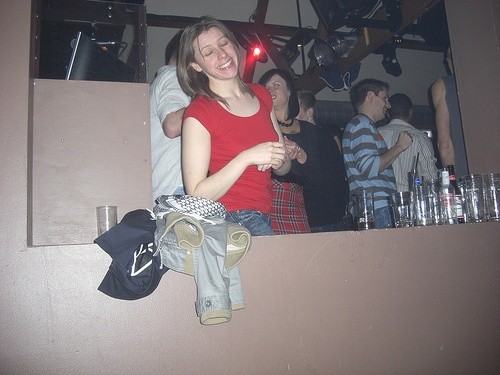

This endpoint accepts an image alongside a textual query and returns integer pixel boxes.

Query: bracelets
[296,145,301,158]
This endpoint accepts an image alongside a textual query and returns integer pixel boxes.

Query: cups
[96,206,118,237]
[353,189,376,231]
[390,173,500,228]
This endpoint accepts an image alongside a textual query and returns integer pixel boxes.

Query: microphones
[70,39,123,49]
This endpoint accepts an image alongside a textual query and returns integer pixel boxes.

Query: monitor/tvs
[65,31,137,82]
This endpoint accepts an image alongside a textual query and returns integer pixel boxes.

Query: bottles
[411,168,422,227]
[448,164,466,223]
[439,171,457,224]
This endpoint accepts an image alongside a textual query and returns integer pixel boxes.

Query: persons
[376,92,438,193]
[431,45,470,182]
[257,69,357,235]
[342,80,413,229]
[148,29,191,211]
[177,14,292,236]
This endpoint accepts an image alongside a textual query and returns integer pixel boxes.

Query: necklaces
[276,118,294,127]
